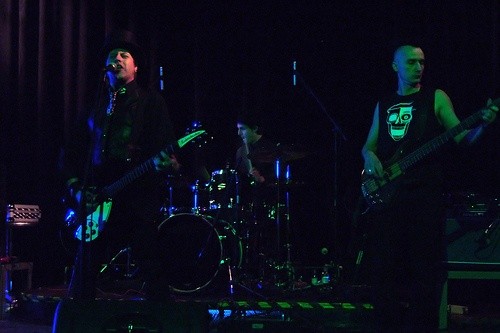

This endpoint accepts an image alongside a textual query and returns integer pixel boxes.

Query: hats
[96,31,147,69]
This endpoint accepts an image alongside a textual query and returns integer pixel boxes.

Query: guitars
[361,102,500,211]
[56,129,206,243]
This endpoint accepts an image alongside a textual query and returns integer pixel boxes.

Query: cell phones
[101,63,117,73]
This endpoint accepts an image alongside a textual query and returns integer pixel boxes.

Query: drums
[156,169,243,214]
[245,196,291,228]
[151,210,242,293]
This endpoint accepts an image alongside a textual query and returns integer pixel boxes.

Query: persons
[64,41,180,299]
[284,140,321,262]
[361,44,498,288]
[225,114,286,253]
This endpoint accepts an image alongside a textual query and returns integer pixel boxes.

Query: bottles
[311,270,318,284]
[322,268,329,288]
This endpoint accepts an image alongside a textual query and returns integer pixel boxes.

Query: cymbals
[262,179,308,187]
[247,144,306,163]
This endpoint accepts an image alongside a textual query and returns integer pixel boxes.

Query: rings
[368,168,374,175]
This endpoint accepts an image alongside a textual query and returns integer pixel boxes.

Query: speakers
[443,218,499,265]
